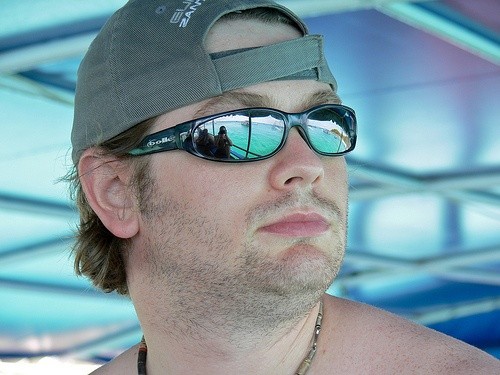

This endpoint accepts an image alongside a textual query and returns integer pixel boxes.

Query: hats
[71,0,339,151]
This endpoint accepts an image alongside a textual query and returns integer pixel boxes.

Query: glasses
[121,104,357,163]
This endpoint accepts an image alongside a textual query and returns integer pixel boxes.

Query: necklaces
[137,296,324,375]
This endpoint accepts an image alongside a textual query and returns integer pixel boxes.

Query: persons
[65,0,500,375]
[196,126,234,160]
